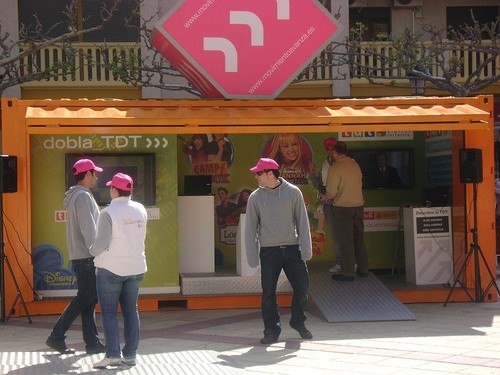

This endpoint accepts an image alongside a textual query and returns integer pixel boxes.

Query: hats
[72,159,103,176]
[249,158,279,173]
[323,138,337,149]
[106,173,134,191]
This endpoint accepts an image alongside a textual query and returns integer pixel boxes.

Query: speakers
[459,148,483,182]
[0,154,18,193]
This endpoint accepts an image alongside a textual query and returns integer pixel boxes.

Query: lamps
[407,64,429,96]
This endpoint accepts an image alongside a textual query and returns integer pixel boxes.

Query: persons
[216,188,238,224]
[233,189,253,214]
[181,134,231,165]
[365,152,401,188]
[89,172,148,367]
[245,158,312,345]
[45,158,104,354]
[269,133,305,169]
[321,138,368,282]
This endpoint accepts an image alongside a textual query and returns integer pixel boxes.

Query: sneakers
[332,274,353,281]
[121,356,136,366]
[93,355,121,368]
[85,340,107,354]
[263,325,281,343]
[46,337,75,354]
[329,264,340,272]
[289,319,312,339]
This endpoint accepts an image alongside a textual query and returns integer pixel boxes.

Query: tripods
[443,183,500,306]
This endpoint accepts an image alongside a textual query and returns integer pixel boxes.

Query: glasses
[257,170,276,176]
